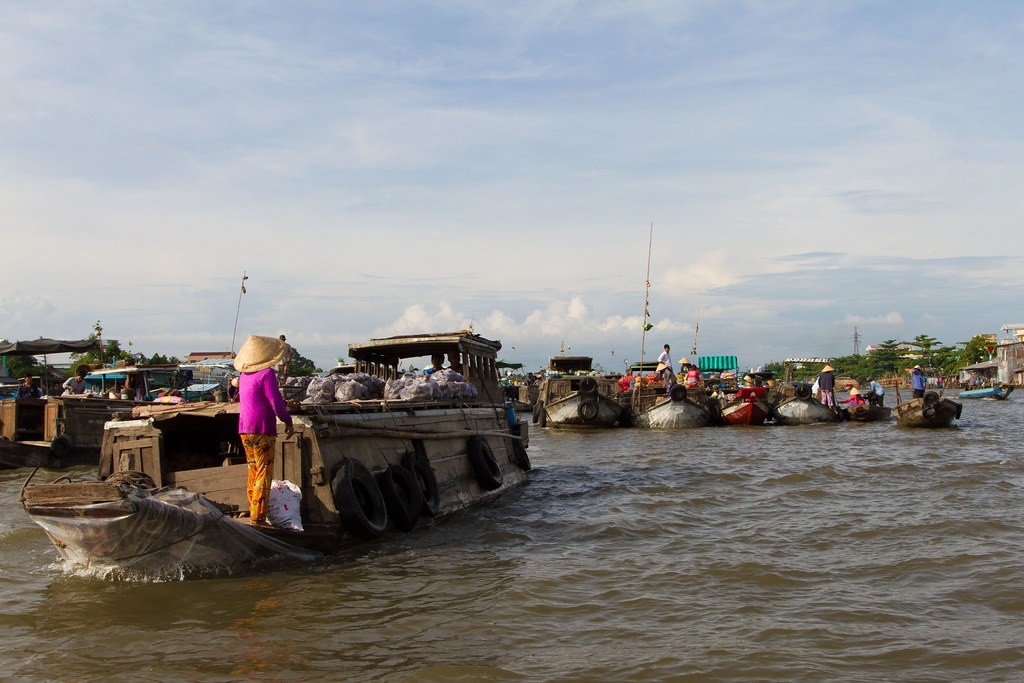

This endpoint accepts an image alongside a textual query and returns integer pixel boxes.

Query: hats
[656,363,668,372]
[821,364,835,372]
[231,377,239,387]
[744,375,751,381]
[865,377,873,382]
[914,365,921,369]
[712,385,718,392]
[850,386,860,396]
[279,335,286,340]
[678,357,690,364]
[234,335,287,372]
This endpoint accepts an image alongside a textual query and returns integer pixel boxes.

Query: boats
[18,330,532,577]
[892,393,963,428]
[0,335,540,465]
[958,360,1014,399]
[538,354,891,429]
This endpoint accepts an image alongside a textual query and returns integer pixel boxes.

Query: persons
[655,344,676,386]
[818,365,835,409]
[61,369,86,398]
[678,357,692,373]
[838,384,866,406]
[524,372,534,385]
[865,377,885,407]
[276,335,293,387]
[234,336,294,526]
[17,374,41,432]
[969,373,976,390]
[418,352,445,376]
[685,365,700,387]
[911,364,926,399]
[446,352,471,376]
[228,377,239,402]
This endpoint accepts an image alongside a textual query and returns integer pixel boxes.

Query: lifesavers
[579,377,597,393]
[578,399,599,420]
[956,404,962,419]
[539,408,546,427]
[333,457,388,539]
[671,384,687,400]
[532,399,544,423]
[923,392,939,403]
[512,432,531,471]
[406,452,440,517]
[923,407,939,421]
[712,405,721,420]
[50,435,72,457]
[795,384,812,401]
[469,433,503,490]
[383,465,422,531]
